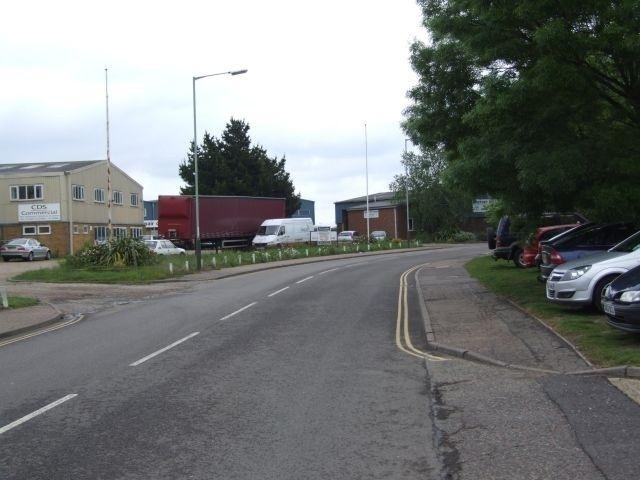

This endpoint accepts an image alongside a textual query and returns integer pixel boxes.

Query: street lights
[192,69,248,272]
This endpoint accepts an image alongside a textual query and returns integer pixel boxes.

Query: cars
[143,235,186,256]
[370,230,390,241]
[487,211,640,336]
[338,231,363,242]
[0,237,51,261]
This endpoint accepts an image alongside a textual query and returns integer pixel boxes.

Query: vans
[251,218,314,250]
